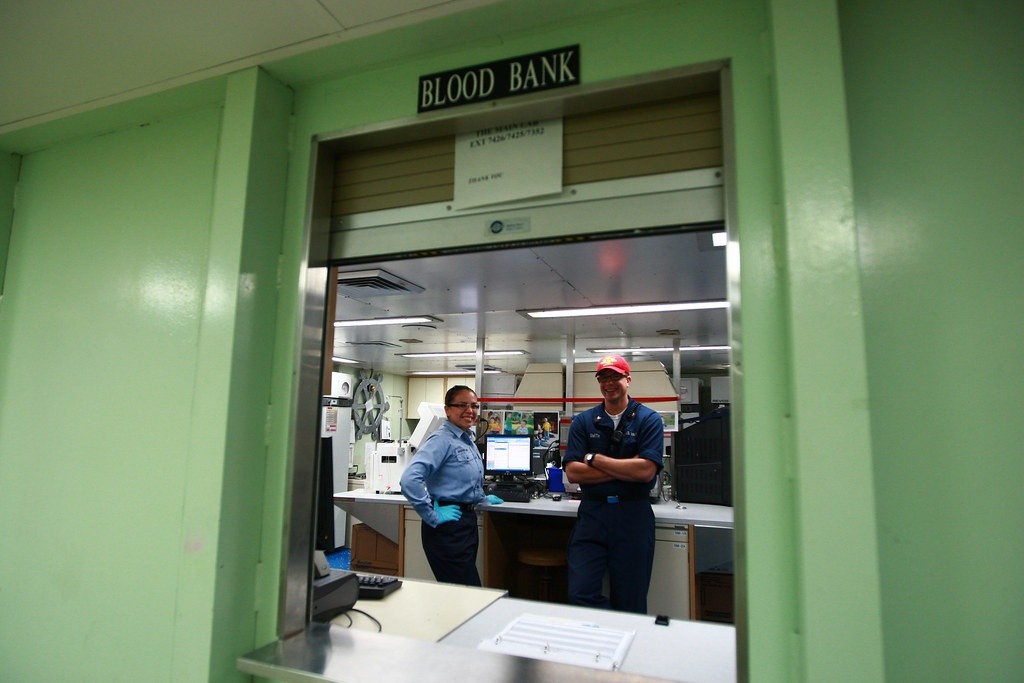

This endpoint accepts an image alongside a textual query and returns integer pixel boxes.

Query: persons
[400,384,486,587]
[505,413,519,435]
[562,355,664,614]
[515,420,528,435]
[483,412,502,434]
[541,417,552,441]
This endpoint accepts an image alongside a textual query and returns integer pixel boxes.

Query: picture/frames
[655,411,678,431]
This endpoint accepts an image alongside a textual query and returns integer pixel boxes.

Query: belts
[583,493,649,505]
[431,500,474,512]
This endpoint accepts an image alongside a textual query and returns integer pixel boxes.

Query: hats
[595,354,630,377]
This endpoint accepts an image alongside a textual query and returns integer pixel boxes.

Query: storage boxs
[350,524,399,576]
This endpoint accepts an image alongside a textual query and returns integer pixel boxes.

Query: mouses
[553,494,562,501]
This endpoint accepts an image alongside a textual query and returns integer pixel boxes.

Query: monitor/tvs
[314,436,336,552]
[483,434,534,483]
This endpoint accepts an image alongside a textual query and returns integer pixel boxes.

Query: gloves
[484,495,504,505]
[433,501,462,524]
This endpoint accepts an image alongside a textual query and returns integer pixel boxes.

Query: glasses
[597,373,627,383]
[448,404,480,410]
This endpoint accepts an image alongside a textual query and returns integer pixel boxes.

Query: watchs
[586,453,596,466]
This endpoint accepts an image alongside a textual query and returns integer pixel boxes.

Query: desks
[332,490,734,620]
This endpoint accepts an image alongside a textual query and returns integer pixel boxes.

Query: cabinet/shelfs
[405,378,443,418]
[444,377,476,405]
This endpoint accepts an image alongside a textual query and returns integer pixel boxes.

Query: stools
[517,546,568,601]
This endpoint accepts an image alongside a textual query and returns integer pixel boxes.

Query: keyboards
[357,576,403,600]
[484,489,530,503]
